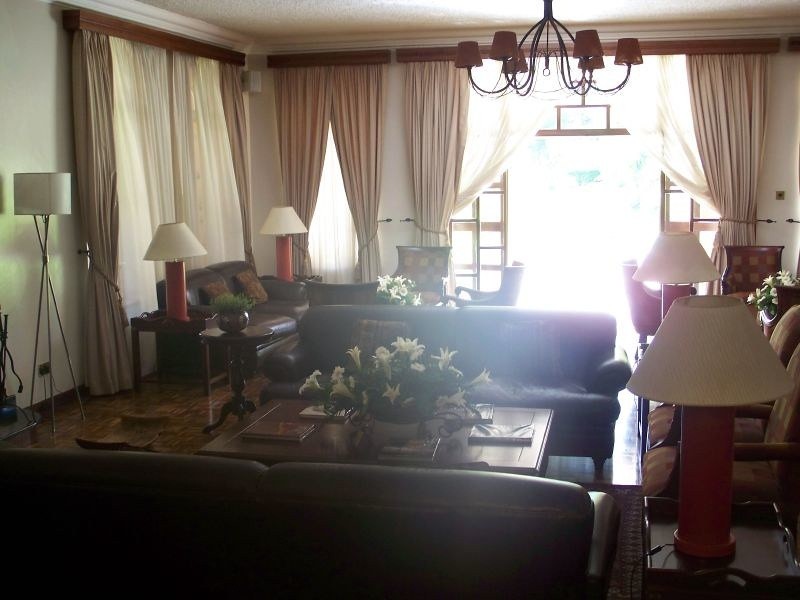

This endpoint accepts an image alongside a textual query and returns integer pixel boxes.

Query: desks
[198,326,274,433]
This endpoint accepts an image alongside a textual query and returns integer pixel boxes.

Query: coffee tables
[197,399,554,477]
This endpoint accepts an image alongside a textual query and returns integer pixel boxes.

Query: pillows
[201,278,229,302]
[350,319,409,363]
[497,314,565,385]
[236,268,267,304]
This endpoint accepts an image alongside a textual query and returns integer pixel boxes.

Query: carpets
[580,483,644,600]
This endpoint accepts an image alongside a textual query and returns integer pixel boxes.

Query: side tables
[644,495,800,600]
[132,317,230,393]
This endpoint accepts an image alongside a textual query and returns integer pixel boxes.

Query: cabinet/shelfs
[449,167,508,287]
[660,169,717,237]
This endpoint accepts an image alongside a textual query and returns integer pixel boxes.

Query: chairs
[641,305,800,533]
[621,258,662,363]
[308,245,525,307]
[721,246,785,294]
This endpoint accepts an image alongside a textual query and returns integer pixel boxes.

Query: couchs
[156,260,309,378]
[0,449,622,600]
[261,306,632,483]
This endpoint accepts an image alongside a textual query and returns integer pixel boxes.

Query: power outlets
[38,361,50,377]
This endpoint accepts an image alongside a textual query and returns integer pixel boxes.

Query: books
[244,417,319,441]
[378,432,441,461]
[468,423,535,442]
[299,405,346,417]
[446,403,494,424]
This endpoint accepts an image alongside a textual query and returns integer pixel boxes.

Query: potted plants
[209,292,254,333]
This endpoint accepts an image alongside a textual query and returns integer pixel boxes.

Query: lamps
[259,207,308,282]
[11,172,87,435]
[624,230,794,558]
[454,0,646,100]
[143,221,208,321]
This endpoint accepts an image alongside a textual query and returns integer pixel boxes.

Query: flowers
[375,274,421,306]
[297,337,493,424]
[745,268,800,317]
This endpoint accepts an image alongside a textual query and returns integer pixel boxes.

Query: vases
[370,416,417,451]
